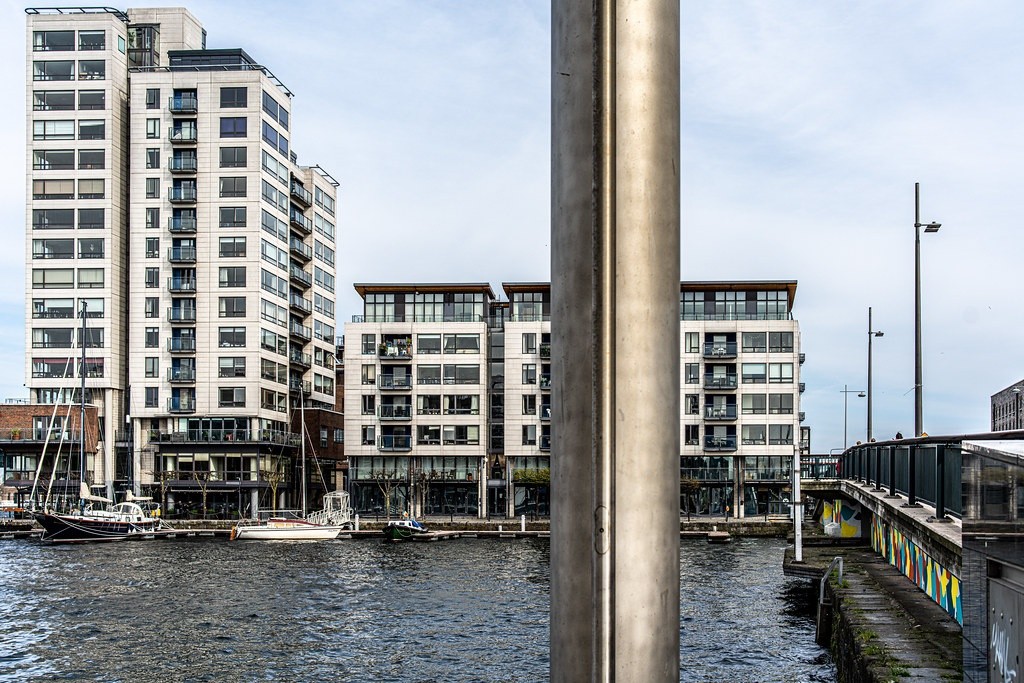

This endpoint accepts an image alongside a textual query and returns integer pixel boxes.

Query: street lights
[865,306,886,441]
[841,383,866,454]
[908,181,944,441]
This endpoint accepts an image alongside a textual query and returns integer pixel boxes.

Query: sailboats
[229,381,343,542]
[22,298,163,546]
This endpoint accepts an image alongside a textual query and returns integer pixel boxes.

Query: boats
[382,517,428,543]
